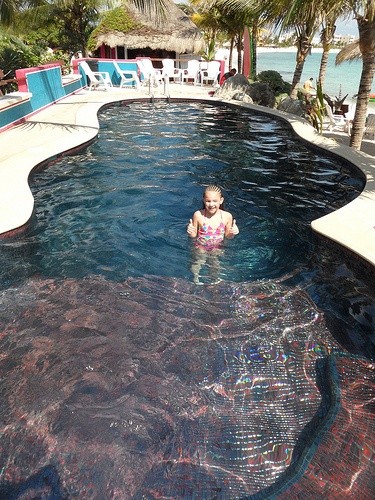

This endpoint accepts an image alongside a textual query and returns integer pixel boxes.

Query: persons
[187,186,240,240]
[303,78,315,91]
[220,68,237,86]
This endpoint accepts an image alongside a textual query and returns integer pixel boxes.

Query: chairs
[137,58,165,86]
[182,60,200,85]
[326,105,375,141]
[200,61,221,87]
[113,60,141,89]
[80,61,113,92]
[161,59,182,85]
